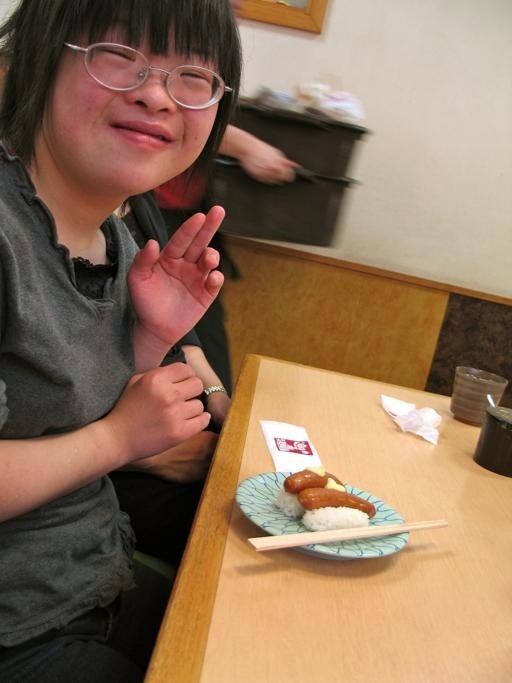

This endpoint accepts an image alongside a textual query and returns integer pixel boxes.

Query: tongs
[301,506,370,532]
[275,490,306,519]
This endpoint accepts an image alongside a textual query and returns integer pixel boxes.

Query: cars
[450,367,509,425]
[470,402,512,477]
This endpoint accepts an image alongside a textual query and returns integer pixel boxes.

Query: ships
[284,470,345,494]
[298,487,376,518]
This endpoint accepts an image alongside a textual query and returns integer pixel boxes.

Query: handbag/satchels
[198,383,229,396]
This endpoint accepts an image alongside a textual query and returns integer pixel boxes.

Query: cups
[62,37,233,113]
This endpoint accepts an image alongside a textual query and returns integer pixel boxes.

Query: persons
[102,188,231,565]
[149,124,303,285]
[1,0,245,681]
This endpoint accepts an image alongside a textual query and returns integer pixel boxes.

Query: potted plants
[232,0,330,36]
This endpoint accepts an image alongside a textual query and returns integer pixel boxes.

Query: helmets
[232,470,411,563]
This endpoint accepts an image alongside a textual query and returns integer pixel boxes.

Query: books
[207,159,356,248]
[217,102,373,175]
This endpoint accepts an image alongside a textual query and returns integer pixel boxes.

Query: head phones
[245,520,449,551]
